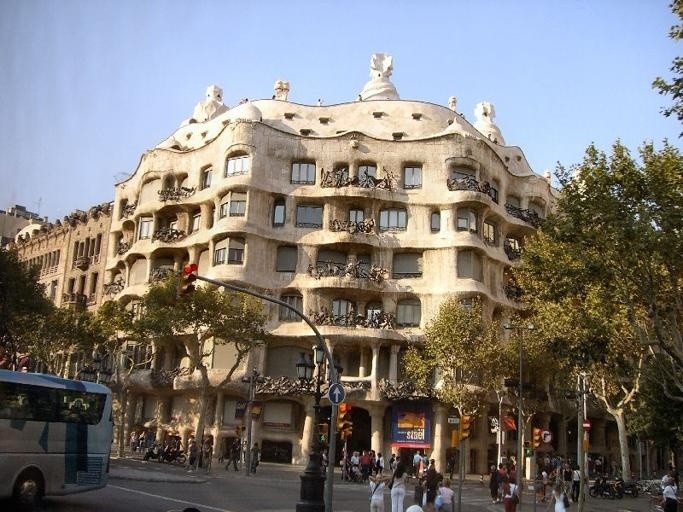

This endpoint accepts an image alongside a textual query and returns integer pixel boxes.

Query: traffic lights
[523,441,533,457]
[342,421,353,437]
[338,402,351,431]
[461,415,473,441]
[533,428,541,448]
[181,263,197,294]
[316,423,327,443]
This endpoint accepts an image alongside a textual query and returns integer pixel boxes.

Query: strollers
[345,462,364,484]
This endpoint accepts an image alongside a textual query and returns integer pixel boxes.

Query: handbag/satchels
[563,493,570,508]
[369,483,379,502]
[512,491,520,505]
[388,470,397,489]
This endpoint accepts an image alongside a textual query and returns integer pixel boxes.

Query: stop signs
[582,421,590,432]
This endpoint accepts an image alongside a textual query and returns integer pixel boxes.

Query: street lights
[502,323,536,511]
[241,366,264,476]
[295,337,343,511]
[562,365,600,496]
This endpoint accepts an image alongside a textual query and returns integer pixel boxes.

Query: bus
[0,369,112,511]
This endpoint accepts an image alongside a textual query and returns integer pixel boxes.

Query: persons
[130,428,261,474]
[660,469,672,489]
[662,477,677,511]
[61,397,100,424]
[320,448,454,511]
[485,451,621,511]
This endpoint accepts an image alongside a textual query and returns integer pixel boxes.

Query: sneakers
[493,499,497,504]
[500,497,504,503]
[496,498,501,504]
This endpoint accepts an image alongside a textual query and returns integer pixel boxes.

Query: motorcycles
[588,471,683,511]
[143,438,187,464]
